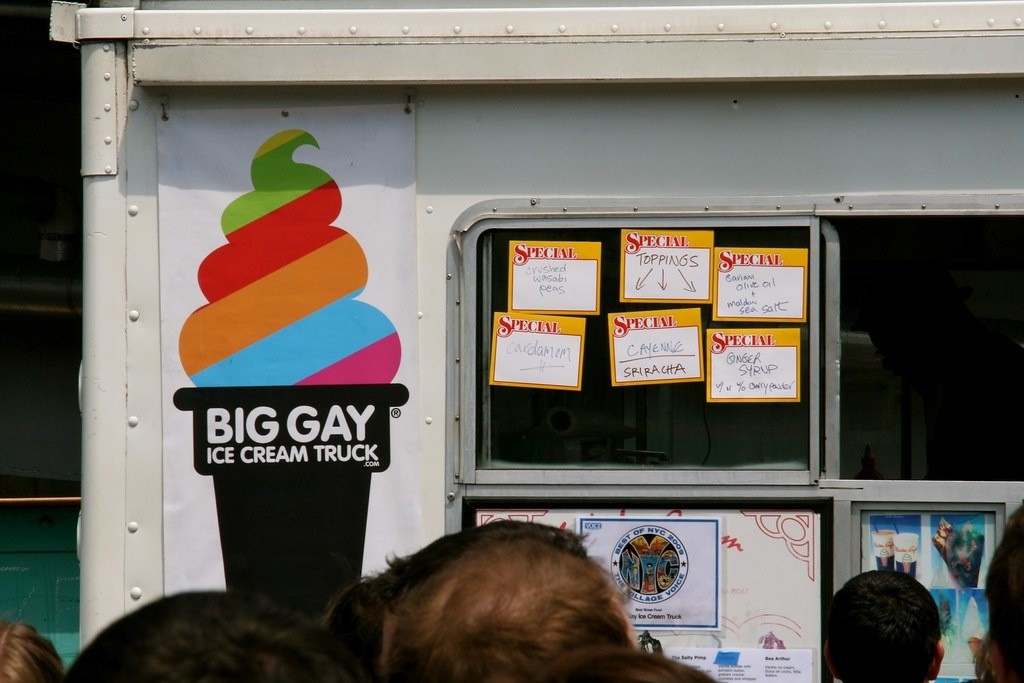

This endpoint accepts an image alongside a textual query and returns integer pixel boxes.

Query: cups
[895,533,918,579]
[872,530,896,572]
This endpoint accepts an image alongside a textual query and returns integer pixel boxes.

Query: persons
[823,570,944,683]
[0,520,720,683]
[964,499,1024,683]
